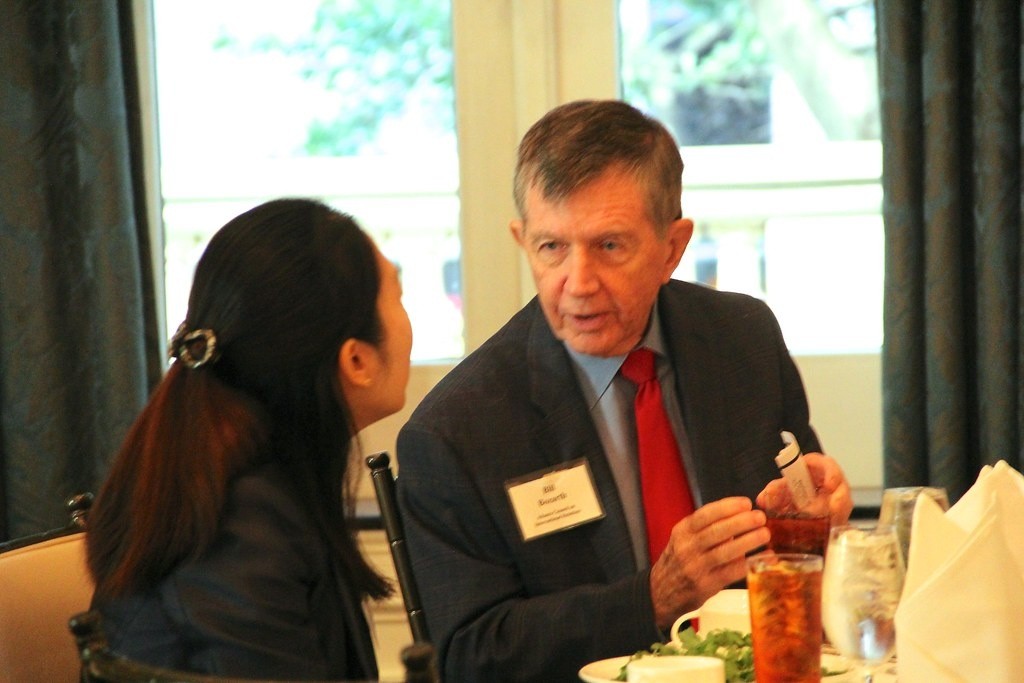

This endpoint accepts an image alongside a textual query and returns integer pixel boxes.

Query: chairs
[0,493,270,683]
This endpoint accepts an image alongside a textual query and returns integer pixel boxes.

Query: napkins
[894,460,1024,682]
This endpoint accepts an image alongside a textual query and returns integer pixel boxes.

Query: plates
[579,654,868,683]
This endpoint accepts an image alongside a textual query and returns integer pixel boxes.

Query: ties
[618,345,700,631]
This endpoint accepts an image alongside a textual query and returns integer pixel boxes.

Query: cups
[627,656,726,683]
[746,550,826,683]
[878,486,949,571]
[669,589,748,653]
[764,482,832,562]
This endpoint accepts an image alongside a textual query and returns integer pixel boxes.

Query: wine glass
[820,521,906,682]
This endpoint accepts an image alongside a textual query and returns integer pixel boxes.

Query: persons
[81,197,399,683]
[395,97,855,683]
